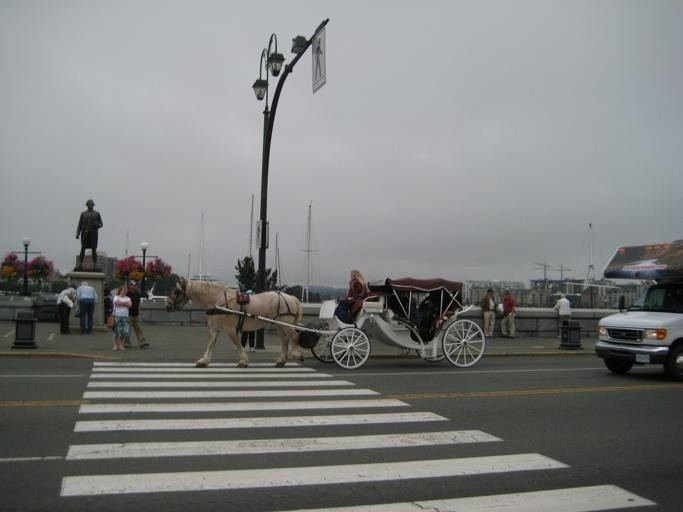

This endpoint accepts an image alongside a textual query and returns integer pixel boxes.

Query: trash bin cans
[557,320,584,350]
[11,310,39,349]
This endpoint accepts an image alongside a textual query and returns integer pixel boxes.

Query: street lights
[252,18,328,350]
[18,236,30,297]
[138,241,148,298]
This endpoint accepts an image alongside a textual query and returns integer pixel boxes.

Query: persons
[552,293,573,338]
[56,283,76,335]
[335,271,368,323]
[120,280,151,350]
[109,287,132,351]
[500,290,516,339]
[239,289,257,353]
[417,296,438,330]
[479,288,498,337]
[73,281,99,335]
[74,200,103,273]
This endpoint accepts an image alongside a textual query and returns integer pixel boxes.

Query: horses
[166,274,304,368]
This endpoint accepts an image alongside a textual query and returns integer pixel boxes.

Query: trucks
[594,238,682,385]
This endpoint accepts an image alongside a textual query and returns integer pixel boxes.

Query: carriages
[164,274,486,370]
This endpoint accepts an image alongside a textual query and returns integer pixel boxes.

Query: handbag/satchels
[107,316,114,328]
[61,294,74,308]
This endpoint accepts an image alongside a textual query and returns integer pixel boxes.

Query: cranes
[532,263,573,294]
[532,261,549,288]
